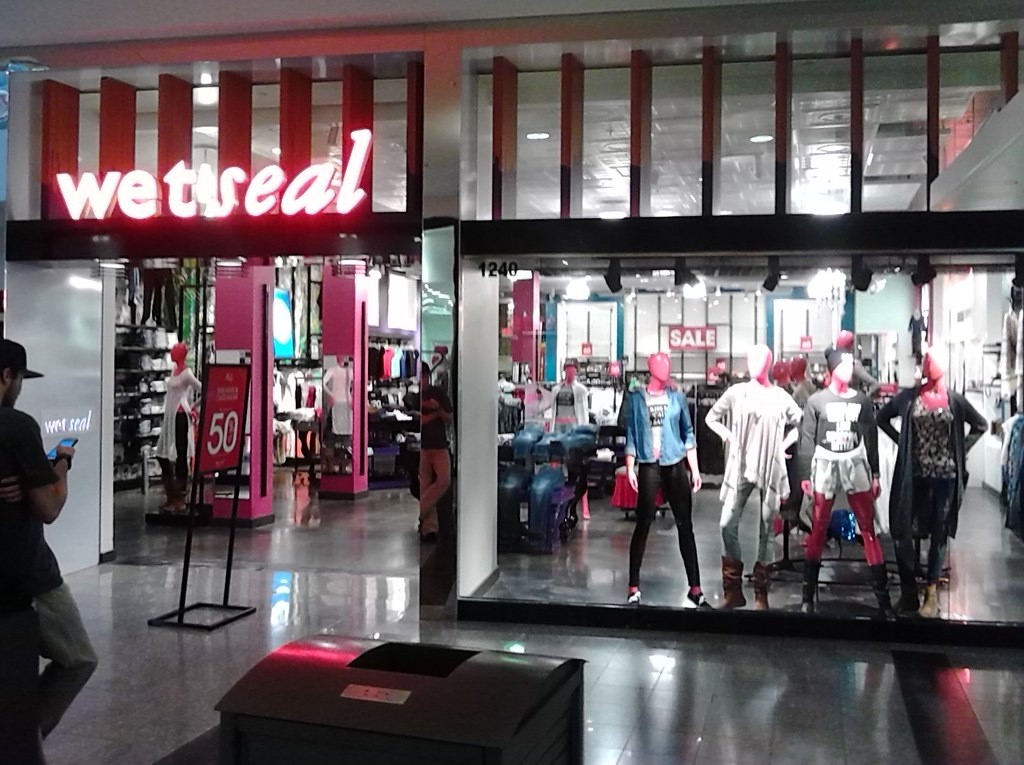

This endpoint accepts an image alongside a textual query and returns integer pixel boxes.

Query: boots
[867,564,898,621]
[753,561,769,610]
[717,555,746,611]
[801,563,820,614]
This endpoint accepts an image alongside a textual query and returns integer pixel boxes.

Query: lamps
[911,254,938,286]
[850,254,873,291]
[604,258,623,293]
[763,257,783,291]
[683,275,707,300]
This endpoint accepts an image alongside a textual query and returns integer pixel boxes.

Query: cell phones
[47,437,79,461]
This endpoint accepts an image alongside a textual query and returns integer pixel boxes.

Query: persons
[420,360,454,538]
[0,338,98,765]
[155,329,987,624]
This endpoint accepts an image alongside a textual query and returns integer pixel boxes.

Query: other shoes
[427,532,437,543]
[628,591,641,605]
[893,580,920,614]
[916,584,939,617]
[687,591,711,607]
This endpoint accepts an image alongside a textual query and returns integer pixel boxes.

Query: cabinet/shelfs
[117,324,198,482]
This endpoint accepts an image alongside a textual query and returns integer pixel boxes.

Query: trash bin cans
[213,631,589,765]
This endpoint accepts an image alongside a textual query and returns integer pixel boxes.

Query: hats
[0,340,44,378]
[824,348,855,373]
[422,362,430,373]
[563,358,580,372]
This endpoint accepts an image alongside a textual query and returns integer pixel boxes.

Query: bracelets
[53,453,72,470]
